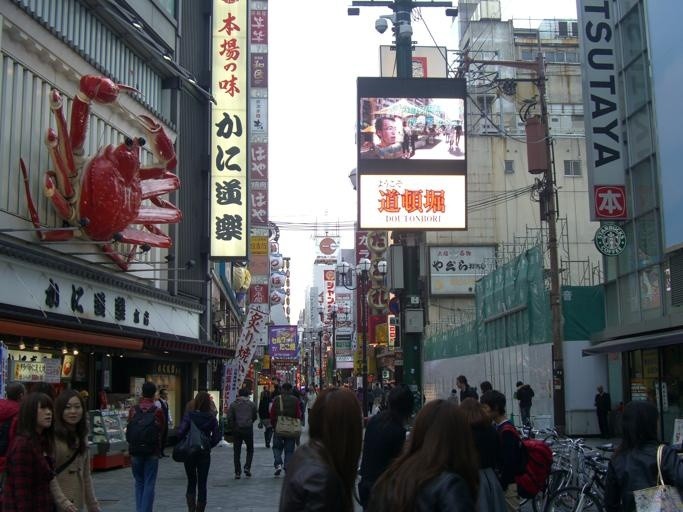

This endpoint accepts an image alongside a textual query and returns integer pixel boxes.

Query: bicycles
[503,409,614,512]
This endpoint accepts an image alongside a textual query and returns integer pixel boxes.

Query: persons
[361,114,463,161]
[1,376,103,512]
[593,385,611,439]
[603,400,683,511]
[277,376,533,512]
[124,378,307,512]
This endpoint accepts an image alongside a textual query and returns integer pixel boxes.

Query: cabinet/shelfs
[83,407,131,471]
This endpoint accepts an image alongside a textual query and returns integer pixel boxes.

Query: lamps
[110,259,198,274]
[27,232,123,246]
[19,337,78,356]
[136,275,214,285]
[92,254,175,267]
[61,243,152,256]
[0,216,91,233]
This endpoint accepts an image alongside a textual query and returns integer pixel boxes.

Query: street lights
[318,303,351,385]
[338,257,388,416]
[302,323,322,394]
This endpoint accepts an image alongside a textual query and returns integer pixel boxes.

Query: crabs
[18,72,184,273]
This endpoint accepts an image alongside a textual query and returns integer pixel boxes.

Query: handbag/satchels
[633,444,683,512]
[172,411,209,462]
[258,422,263,428]
[275,415,301,439]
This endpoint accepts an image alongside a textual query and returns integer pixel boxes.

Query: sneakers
[274,464,282,475]
[235,472,240,478]
[244,465,251,476]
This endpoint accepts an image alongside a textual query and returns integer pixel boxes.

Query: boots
[187,494,196,512]
[196,500,206,512]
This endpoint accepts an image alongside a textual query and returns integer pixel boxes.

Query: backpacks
[125,405,159,457]
[259,400,272,419]
[497,421,553,499]
[0,417,12,456]
